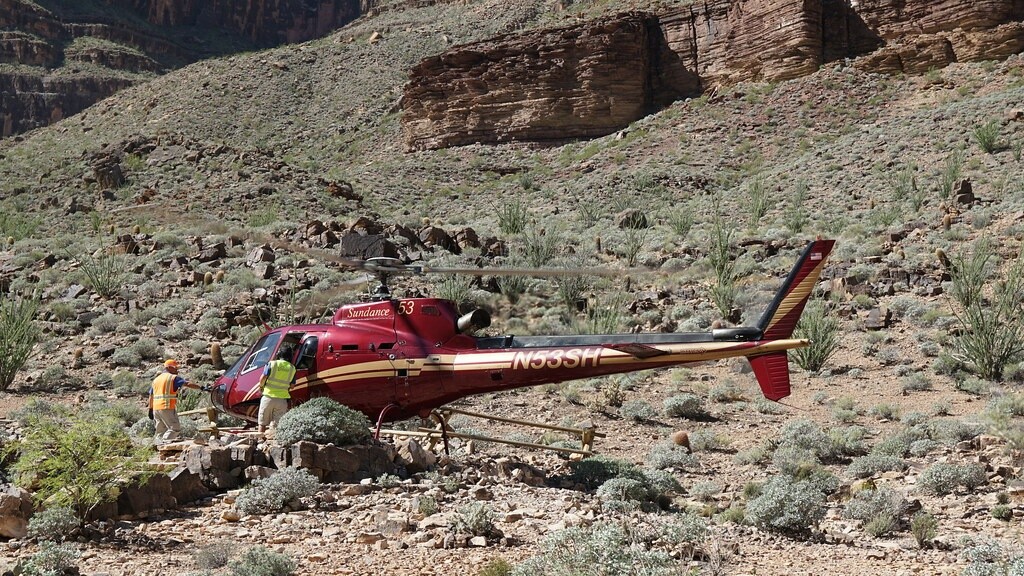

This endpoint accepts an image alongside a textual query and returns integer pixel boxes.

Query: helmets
[165,359,182,368]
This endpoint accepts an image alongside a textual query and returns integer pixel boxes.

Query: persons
[148,359,212,434]
[257,345,296,433]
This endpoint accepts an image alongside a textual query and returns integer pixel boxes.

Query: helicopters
[129,204,835,455]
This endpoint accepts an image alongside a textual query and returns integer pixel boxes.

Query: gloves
[148,409,154,420]
[200,385,212,392]
